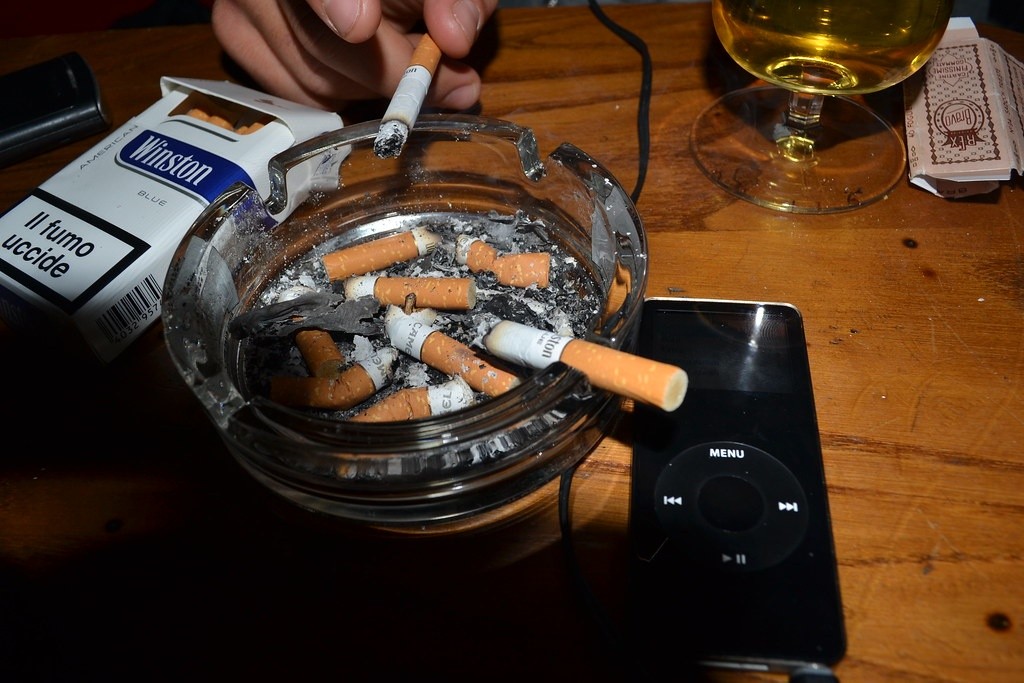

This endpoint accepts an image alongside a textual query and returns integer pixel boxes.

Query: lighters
[1,52,112,170]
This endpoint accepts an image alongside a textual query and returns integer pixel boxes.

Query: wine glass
[688,1,951,214]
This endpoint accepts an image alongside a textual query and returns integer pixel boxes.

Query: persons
[212,0,498,111]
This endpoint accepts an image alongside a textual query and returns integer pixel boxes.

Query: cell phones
[0,52,111,167]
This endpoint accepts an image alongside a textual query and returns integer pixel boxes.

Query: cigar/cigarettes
[483,321,688,413]
[374,32,443,160]
[344,377,474,421]
[321,224,442,281]
[390,315,521,398]
[343,277,478,310]
[291,316,345,375]
[268,348,398,410]
[456,233,551,290]
[168,74,276,136]
